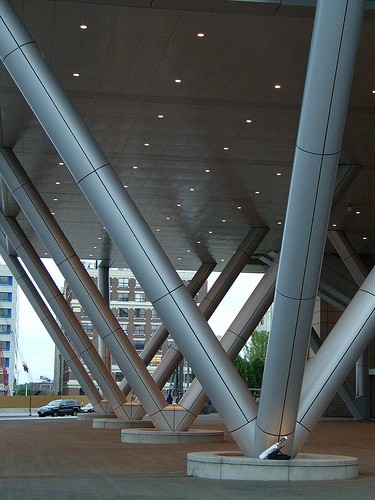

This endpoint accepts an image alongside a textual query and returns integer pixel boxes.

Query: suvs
[37,399,80,416]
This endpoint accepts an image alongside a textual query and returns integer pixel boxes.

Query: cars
[79,402,94,413]
